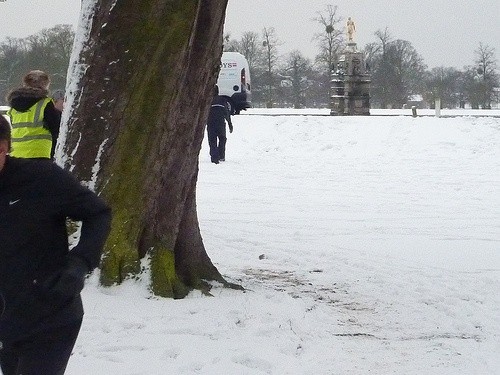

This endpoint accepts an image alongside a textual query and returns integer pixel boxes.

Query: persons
[207,84,234,165]
[6,70,67,164]
[0,114,113,375]
[347,16,356,42]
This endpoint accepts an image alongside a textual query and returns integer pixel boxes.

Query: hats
[23,70,50,90]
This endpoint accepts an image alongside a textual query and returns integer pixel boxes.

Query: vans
[217,50,254,115]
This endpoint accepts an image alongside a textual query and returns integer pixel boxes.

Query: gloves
[229,124,233,133]
[32,252,91,301]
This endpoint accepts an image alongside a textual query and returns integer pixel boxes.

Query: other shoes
[211,158,218,162]
[220,157,225,161]
